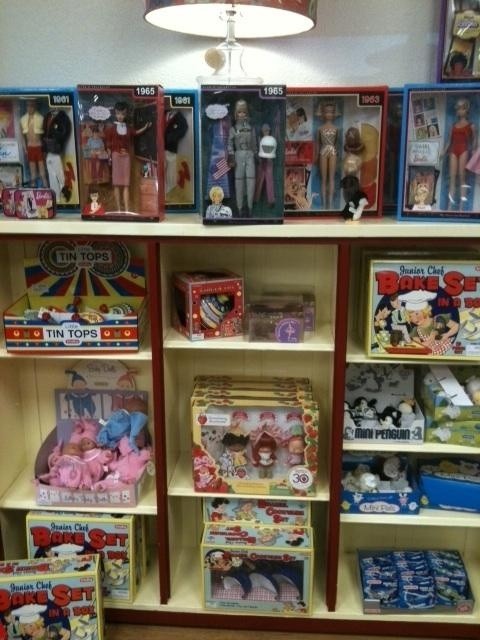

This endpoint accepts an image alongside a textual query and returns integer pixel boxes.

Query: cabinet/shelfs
[0,220,479,640]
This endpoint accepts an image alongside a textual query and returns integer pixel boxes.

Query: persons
[220,427,305,479]
[20,96,477,216]
[343,454,407,494]
[39,395,151,492]
[21,98,477,492]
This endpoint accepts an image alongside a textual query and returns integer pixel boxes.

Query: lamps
[142,0,314,89]
